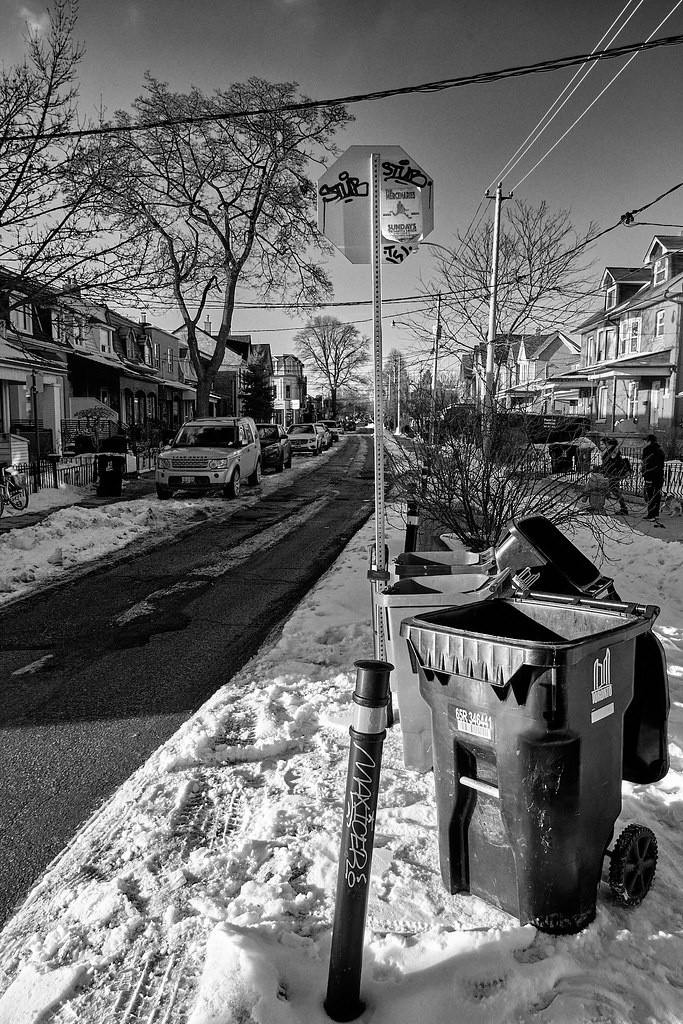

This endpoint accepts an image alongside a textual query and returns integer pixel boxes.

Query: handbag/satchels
[606,448,632,479]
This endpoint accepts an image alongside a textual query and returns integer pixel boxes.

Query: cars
[311,416,374,451]
[254,423,292,473]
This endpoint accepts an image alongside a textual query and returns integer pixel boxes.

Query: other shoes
[615,509,660,522]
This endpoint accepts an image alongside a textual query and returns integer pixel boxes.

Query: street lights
[412,242,513,476]
[390,321,442,450]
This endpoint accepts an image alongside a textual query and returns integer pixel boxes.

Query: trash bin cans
[486,512,625,613]
[385,546,497,588]
[397,588,671,935]
[547,443,579,474]
[96,454,126,497]
[573,447,595,474]
[372,566,516,774]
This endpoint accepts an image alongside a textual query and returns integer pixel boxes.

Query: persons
[583,465,613,515]
[638,434,665,522]
[598,437,632,516]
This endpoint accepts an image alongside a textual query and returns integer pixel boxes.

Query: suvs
[285,423,323,455]
[155,416,261,499]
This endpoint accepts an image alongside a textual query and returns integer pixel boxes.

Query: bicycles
[0,462,29,517]
